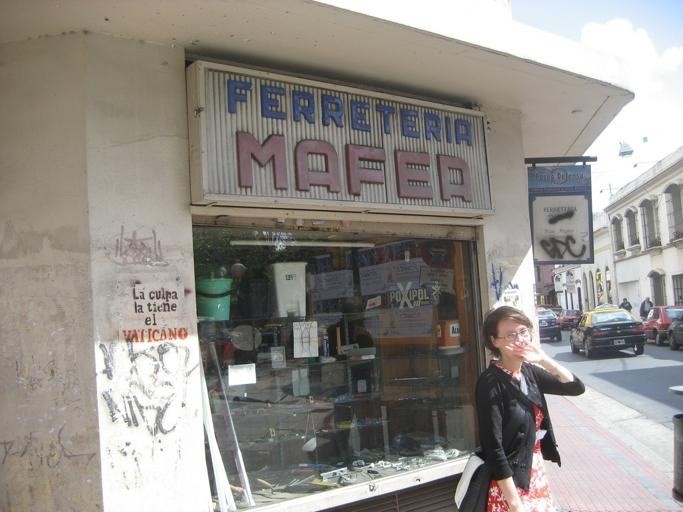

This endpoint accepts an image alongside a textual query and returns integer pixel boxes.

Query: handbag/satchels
[453,453,490,510]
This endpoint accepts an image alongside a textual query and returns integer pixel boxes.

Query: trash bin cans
[668,385,683,502]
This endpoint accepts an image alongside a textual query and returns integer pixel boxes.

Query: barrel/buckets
[195,278,233,321]
[434,319,461,350]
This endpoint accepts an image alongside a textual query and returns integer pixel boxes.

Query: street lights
[599,182,613,195]
[631,160,657,168]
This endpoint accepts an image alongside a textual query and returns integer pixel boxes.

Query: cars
[537,301,683,349]
[566,303,647,358]
[260,323,283,345]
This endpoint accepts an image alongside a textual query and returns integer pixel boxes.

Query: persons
[639,297,652,319]
[619,298,632,311]
[476,306,586,512]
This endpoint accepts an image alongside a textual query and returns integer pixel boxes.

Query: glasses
[495,327,529,341]
